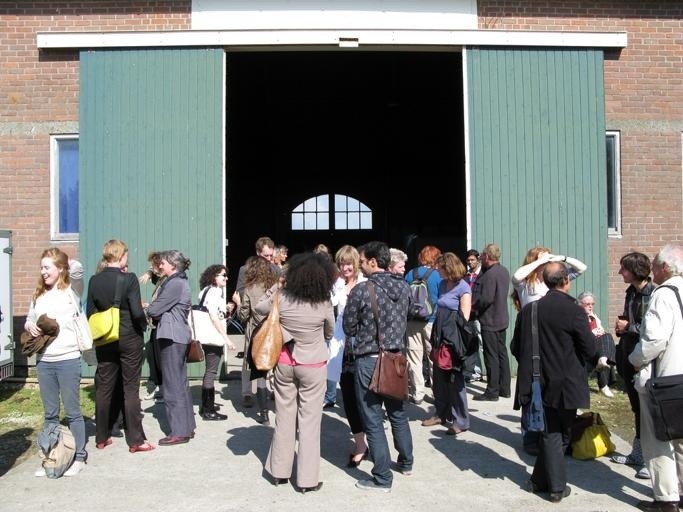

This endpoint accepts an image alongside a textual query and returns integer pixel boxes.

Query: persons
[343,243,416,491]
[26,248,92,477]
[272,244,288,267]
[387,248,406,276]
[137,252,166,404]
[464,250,487,382]
[477,247,510,401]
[421,252,472,437]
[404,245,443,403]
[197,264,235,420]
[147,251,196,445]
[235,256,278,425]
[97,254,129,438]
[255,252,335,494]
[510,262,598,503]
[512,248,587,456]
[575,292,616,399]
[324,246,369,408]
[235,238,283,406]
[87,238,157,451]
[612,254,653,481]
[627,247,683,511]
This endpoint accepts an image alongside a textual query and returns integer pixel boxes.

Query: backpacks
[408,264,436,320]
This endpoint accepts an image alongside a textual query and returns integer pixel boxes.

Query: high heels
[271,474,288,487]
[298,483,325,492]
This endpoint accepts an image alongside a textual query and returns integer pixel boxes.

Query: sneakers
[241,396,252,409]
[144,388,159,399]
[36,466,45,477]
[63,460,87,480]
[356,476,394,490]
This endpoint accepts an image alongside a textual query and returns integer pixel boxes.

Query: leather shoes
[323,401,336,409]
[547,486,572,505]
[599,384,615,400]
[397,463,413,475]
[348,445,370,468]
[422,415,445,427]
[128,444,154,453]
[159,435,189,446]
[595,361,609,376]
[446,426,464,436]
[473,393,500,402]
[96,441,113,449]
[525,480,547,494]
[635,499,678,512]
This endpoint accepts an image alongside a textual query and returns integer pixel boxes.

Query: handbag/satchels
[252,291,282,373]
[429,342,453,371]
[367,281,409,402]
[570,412,616,461]
[186,282,206,364]
[189,285,226,348]
[67,281,94,354]
[644,284,683,442]
[88,272,124,345]
[37,423,75,480]
[522,299,545,434]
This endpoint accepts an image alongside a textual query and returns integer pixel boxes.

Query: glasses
[583,301,596,306]
[215,273,228,278]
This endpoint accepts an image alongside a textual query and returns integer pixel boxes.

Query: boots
[637,468,648,478]
[613,436,646,465]
[203,388,228,421]
[256,387,271,427]
[199,388,221,413]
